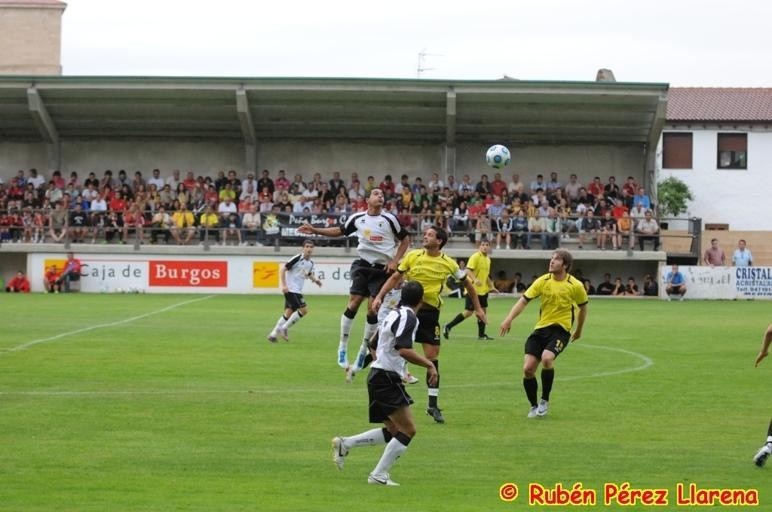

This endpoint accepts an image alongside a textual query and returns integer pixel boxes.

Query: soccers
[486,144,511,168]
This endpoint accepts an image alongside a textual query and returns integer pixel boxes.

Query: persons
[0,168,689,302]
[704,238,726,266]
[499,249,590,418]
[266,238,323,343]
[369,224,490,423]
[443,238,498,341]
[731,239,753,267]
[331,280,440,486]
[753,321,772,468]
[346,274,419,385]
[297,187,411,370]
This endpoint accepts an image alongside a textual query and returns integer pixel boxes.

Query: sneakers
[332,436,350,471]
[338,345,348,368]
[752,443,772,468]
[355,349,367,371]
[367,472,401,487]
[526,406,538,418]
[278,323,289,342]
[443,322,451,340]
[266,334,277,342]
[402,373,419,384]
[478,334,494,340]
[345,363,355,384]
[424,406,445,423]
[536,397,549,417]
